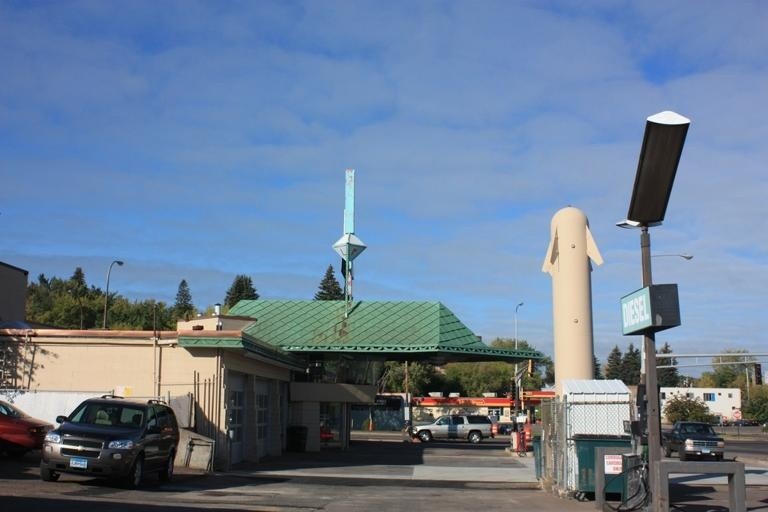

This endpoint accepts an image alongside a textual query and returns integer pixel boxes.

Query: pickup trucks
[661,422,725,461]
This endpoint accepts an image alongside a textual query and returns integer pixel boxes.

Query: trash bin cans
[287,426,308,453]
[567,434,633,501]
[533,436,541,480]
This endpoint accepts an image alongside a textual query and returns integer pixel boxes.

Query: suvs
[412,415,492,444]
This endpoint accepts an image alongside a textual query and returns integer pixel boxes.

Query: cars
[0,395,180,489]
[500,424,512,435]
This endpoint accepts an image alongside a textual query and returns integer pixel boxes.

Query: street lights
[616,111,690,492]
[67,289,83,330]
[104,260,124,329]
[514,301,523,417]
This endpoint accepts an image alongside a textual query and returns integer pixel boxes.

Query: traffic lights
[528,358,536,376]
